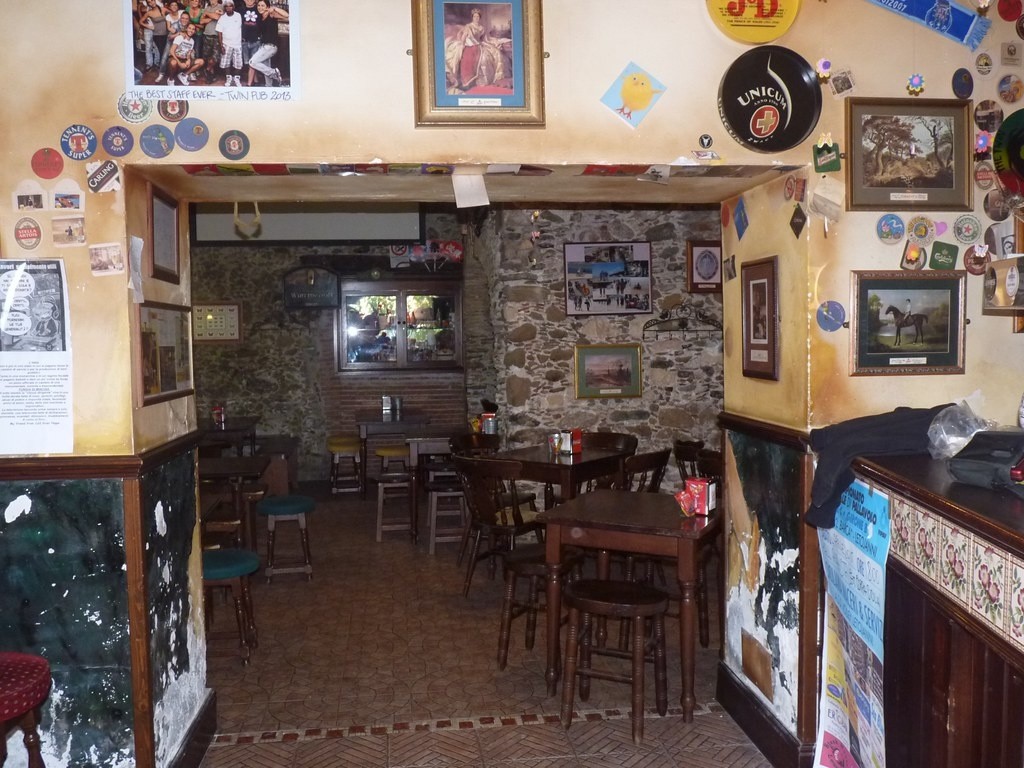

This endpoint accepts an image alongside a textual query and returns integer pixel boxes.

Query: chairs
[441,429,724,648]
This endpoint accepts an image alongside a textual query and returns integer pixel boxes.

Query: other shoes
[225,75,233,86]
[189,73,197,81]
[145,65,151,72]
[154,73,164,82]
[206,74,215,83]
[233,75,242,87]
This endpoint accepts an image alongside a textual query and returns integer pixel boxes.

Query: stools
[497,543,587,672]
[425,479,475,557]
[200,550,259,668]
[326,434,365,494]
[373,444,409,471]
[375,472,418,543]
[0,651,52,768]
[558,578,672,744]
[204,508,246,550]
[223,481,266,550]
[256,494,314,579]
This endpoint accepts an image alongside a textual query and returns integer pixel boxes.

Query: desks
[534,485,725,723]
[405,426,476,545]
[487,439,627,528]
[196,416,261,457]
[199,454,270,552]
[352,414,434,499]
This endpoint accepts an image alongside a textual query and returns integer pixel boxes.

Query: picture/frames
[134,300,195,407]
[847,270,968,376]
[843,96,973,211]
[739,255,780,382]
[574,343,642,400]
[685,239,722,294]
[411,0,546,131]
[562,239,653,315]
[146,180,181,285]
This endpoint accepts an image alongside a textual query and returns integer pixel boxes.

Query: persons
[132,0,289,87]
[28,199,33,207]
[456,8,484,90]
[65,226,73,240]
[347,314,382,363]
[97,258,108,270]
[112,254,120,268]
[568,246,649,311]
[903,298,912,327]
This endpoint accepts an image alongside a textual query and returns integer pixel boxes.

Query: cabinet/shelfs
[851,446,1024,766]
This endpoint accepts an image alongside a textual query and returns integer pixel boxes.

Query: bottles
[548,428,561,455]
[559,429,573,456]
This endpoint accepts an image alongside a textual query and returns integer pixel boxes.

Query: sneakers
[272,68,282,84]
[178,72,190,85]
[167,77,175,86]
